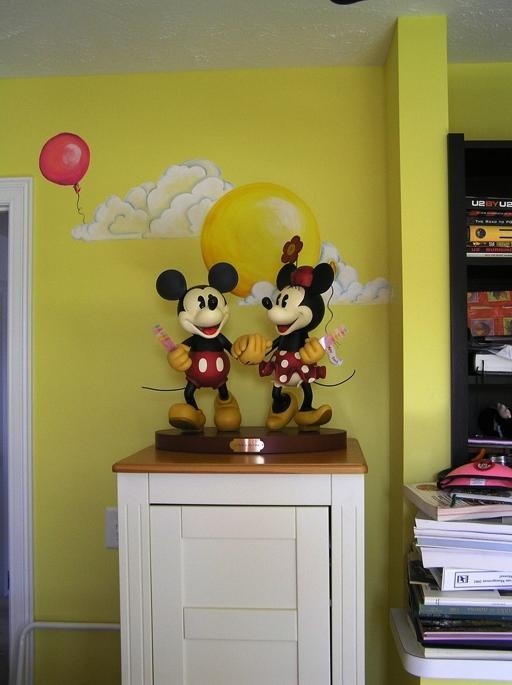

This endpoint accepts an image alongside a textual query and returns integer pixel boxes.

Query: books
[387,482,512,659]
[462,197,512,259]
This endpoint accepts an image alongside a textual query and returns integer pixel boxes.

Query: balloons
[198,182,322,298]
[37,134,94,192]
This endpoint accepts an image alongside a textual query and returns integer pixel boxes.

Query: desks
[389,605,512,685]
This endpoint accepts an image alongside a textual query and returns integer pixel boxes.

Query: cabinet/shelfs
[116,472,365,685]
[449,133,512,468]
